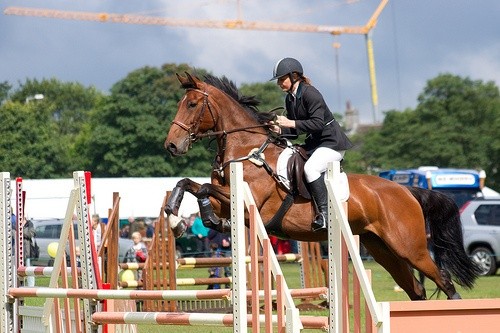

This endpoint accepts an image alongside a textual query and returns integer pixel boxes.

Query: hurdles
[0,160,305,333]
[4,146,391,333]
[96,190,330,313]
[14,177,109,333]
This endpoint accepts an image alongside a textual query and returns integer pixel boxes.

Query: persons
[263,57,355,230]
[10,207,41,286]
[91,209,232,290]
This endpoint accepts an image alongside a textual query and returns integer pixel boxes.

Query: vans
[377,167,485,210]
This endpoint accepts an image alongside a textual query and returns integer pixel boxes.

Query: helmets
[269,57,303,81]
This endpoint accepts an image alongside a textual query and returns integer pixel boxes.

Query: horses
[162,71,486,301]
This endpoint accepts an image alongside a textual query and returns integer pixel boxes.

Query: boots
[308,175,329,228]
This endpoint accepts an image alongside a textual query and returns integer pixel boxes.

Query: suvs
[457,197,500,276]
[24,218,150,267]
